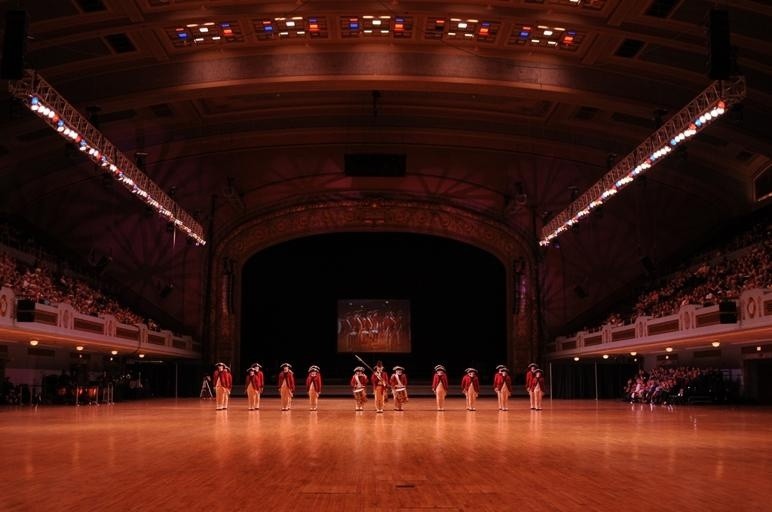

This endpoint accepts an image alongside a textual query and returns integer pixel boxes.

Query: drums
[353,389,364,400]
[395,387,408,400]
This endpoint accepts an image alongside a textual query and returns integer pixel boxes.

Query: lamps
[537,72,749,248]
[11,69,207,248]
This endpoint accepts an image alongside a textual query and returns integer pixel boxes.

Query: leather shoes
[375,407,383,413]
[354,407,364,410]
[498,407,509,410]
[248,406,259,409]
[529,406,542,411]
[279,406,291,411]
[437,406,445,410]
[215,406,228,410]
[467,406,476,411]
[310,406,318,411]
[393,406,404,410]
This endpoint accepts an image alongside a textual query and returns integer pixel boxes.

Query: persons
[278,363,295,411]
[623,367,701,405]
[246,363,264,409]
[213,363,232,410]
[306,365,321,410]
[337,309,401,351]
[606,222,772,327]
[351,360,407,411]
[461,368,479,411]
[494,365,512,410]
[0,251,161,325]
[526,364,545,410]
[432,365,449,410]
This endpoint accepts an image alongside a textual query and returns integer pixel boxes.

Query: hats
[353,360,405,372]
[309,365,319,372]
[280,362,291,368]
[527,363,543,375]
[435,362,447,372]
[496,364,509,372]
[216,361,230,371]
[246,362,262,373]
[465,367,477,374]
